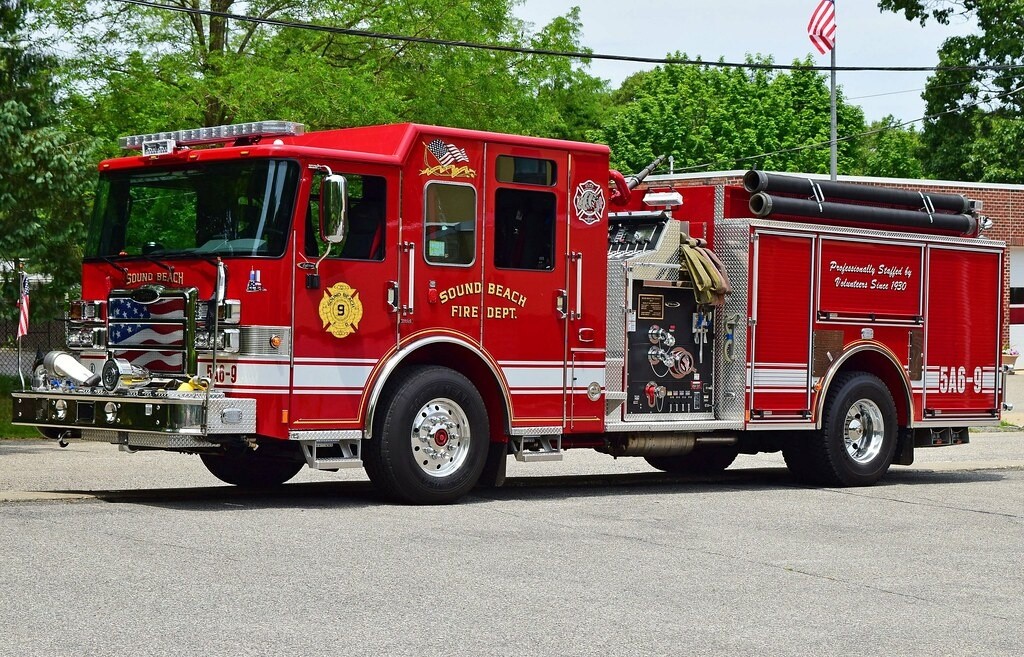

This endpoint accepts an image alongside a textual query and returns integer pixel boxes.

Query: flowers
[1002,349,1019,355]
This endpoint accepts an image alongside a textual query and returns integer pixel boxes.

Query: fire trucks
[11,119,1016,507]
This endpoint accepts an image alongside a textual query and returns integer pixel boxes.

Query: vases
[1002,356,1019,365]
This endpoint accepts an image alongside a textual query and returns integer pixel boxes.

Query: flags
[17,270,30,340]
[807,0,837,54]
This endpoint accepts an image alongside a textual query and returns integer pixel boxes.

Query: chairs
[337,201,385,262]
